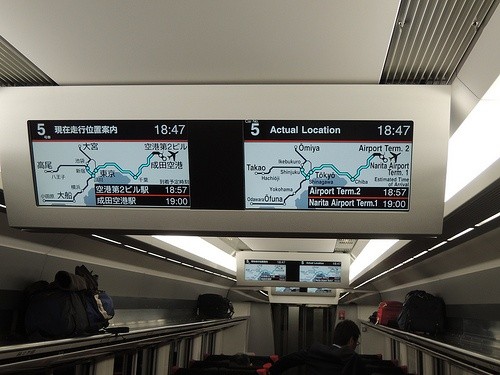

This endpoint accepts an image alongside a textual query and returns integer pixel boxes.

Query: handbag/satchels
[16,265,115,340]
[196,293,234,320]
[375,301,404,326]
[395,290,446,336]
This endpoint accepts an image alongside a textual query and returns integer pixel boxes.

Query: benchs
[172,354,279,375]
[359,352,412,375]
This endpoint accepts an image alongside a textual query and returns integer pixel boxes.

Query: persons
[310,319,361,362]
